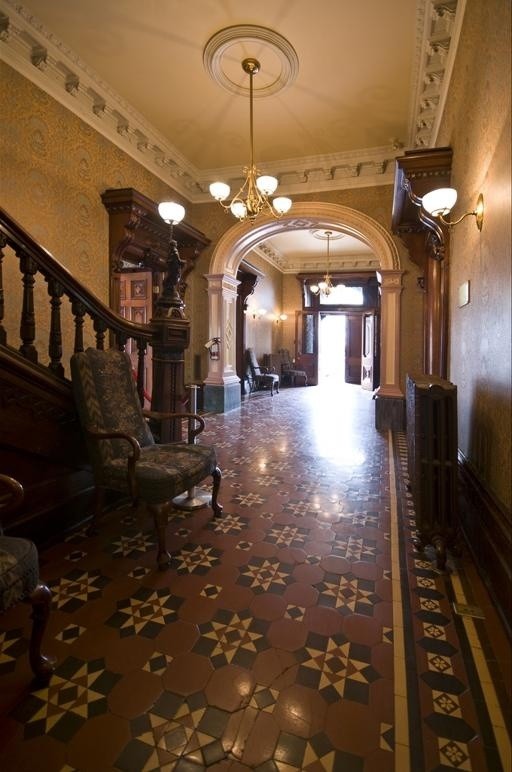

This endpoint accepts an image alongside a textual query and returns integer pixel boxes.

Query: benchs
[373,383,404,431]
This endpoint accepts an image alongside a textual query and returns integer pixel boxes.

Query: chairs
[247,348,309,396]
[71,347,224,568]
[0,474,56,682]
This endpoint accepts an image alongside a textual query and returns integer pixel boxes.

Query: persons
[164,240,188,298]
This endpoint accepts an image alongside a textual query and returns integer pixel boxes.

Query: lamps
[154,201,188,319]
[209,53,293,225]
[309,232,346,297]
[421,188,484,232]
